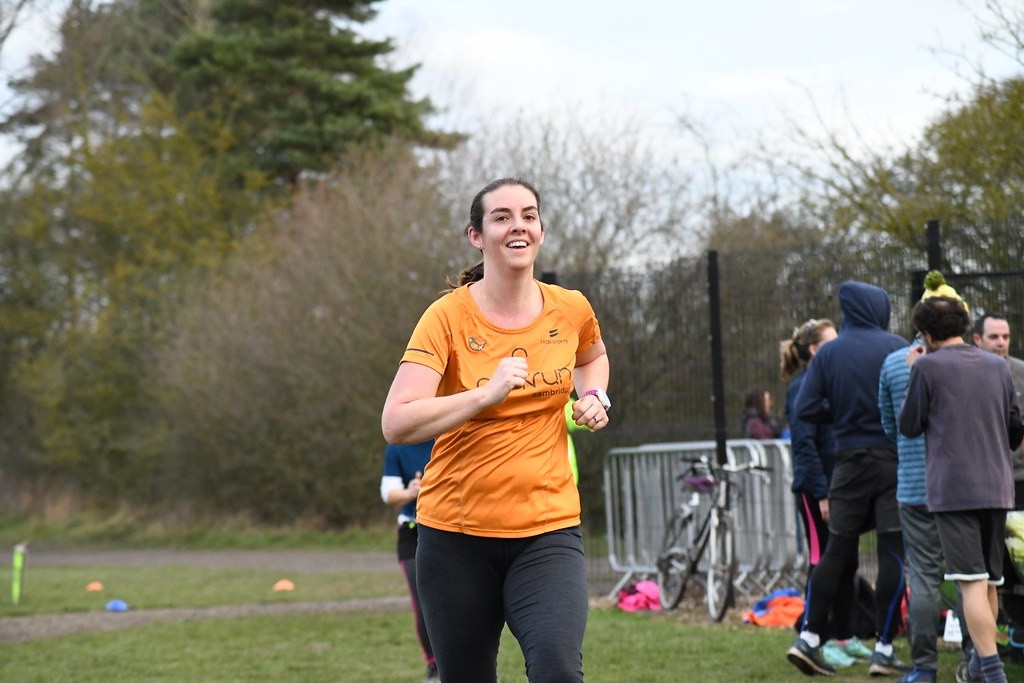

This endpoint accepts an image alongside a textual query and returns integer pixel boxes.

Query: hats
[921,270,969,318]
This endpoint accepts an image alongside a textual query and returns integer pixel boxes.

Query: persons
[876,283,1007,682]
[894,294,1024,682]
[380,439,440,683]
[786,278,912,678]
[966,309,1024,666]
[739,388,781,442]
[777,317,872,674]
[378,176,617,682]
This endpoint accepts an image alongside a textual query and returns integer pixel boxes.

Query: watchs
[578,388,612,412]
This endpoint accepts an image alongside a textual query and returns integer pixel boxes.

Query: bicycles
[655,456,774,622]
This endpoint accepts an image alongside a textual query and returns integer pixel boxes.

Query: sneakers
[425,661,441,682]
[867,653,913,678]
[821,647,857,669]
[898,672,936,682]
[956,661,974,683]
[825,640,876,658]
[784,639,836,676]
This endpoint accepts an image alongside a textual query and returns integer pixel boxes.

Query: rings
[593,416,599,423]
